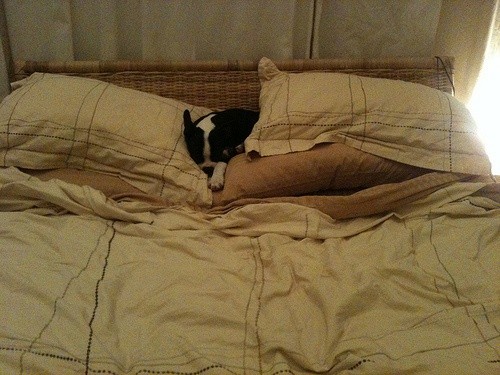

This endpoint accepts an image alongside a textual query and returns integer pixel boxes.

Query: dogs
[182,108,260,193]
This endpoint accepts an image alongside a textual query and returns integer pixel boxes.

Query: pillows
[0,71,216,207]
[244,55,494,179]
[25,166,148,201]
[220,141,430,207]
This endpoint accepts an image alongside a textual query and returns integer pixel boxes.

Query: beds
[0,54,500,375]
